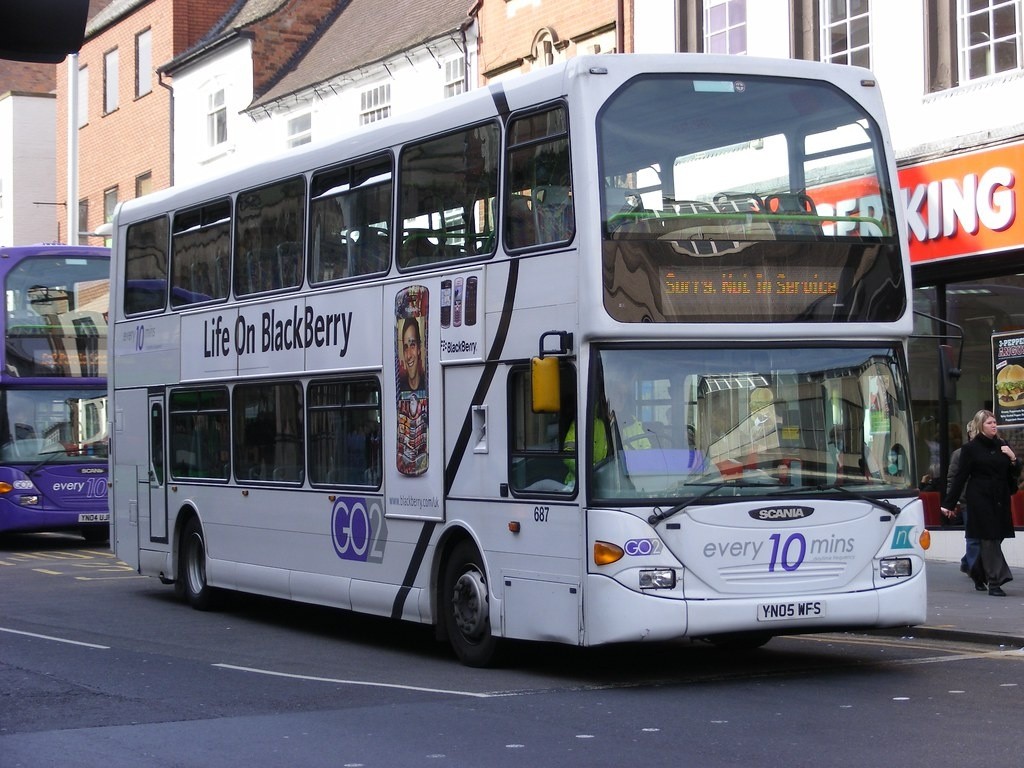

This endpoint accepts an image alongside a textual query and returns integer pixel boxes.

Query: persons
[916,414,940,490]
[563,374,652,485]
[399,318,426,391]
[941,409,1024,597]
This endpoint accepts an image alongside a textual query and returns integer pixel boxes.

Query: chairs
[185,190,824,301]
[224,462,376,486]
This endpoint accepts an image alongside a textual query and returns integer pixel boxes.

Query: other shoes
[975,583,987,591]
[960,565,967,573]
[989,587,1006,596]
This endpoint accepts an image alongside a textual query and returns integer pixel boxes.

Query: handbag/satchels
[1010,478,1019,495]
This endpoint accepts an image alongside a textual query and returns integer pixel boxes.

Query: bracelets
[1011,458,1016,462]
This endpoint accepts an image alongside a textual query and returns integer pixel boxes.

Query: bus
[0,245,113,550]
[109,52,965,670]
